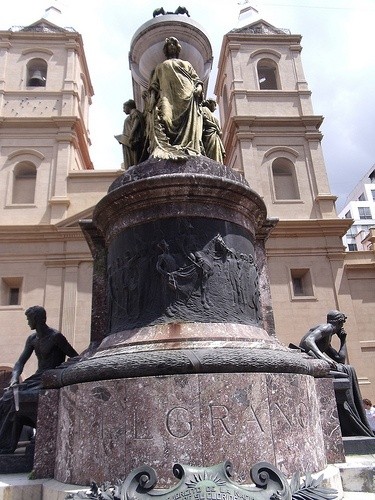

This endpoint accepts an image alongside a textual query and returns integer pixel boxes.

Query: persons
[299,312,370,434]
[106,223,260,330]
[201,98,227,164]
[142,36,203,158]
[363,399,375,436]
[121,99,144,173]
[0,305,79,452]
